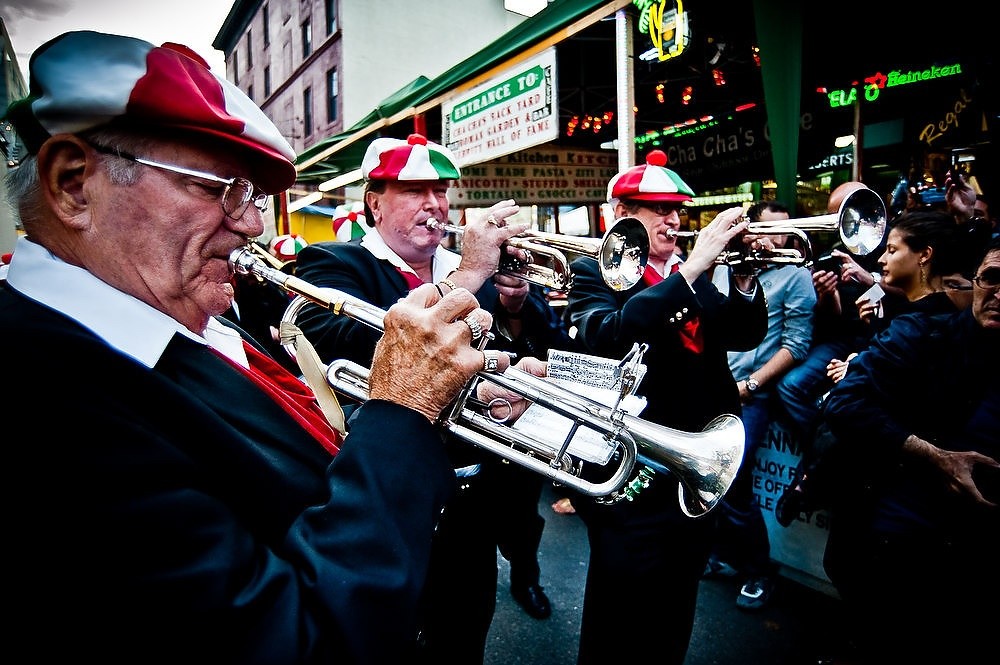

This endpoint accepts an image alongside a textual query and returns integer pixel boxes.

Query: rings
[465,317,482,343]
[487,214,498,225]
[481,349,499,372]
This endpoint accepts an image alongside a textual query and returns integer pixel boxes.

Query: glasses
[624,200,691,217]
[972,274,1000,290]
[85,141,270,221]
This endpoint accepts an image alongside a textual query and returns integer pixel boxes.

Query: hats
[360,133,462,181]
[6,31,298,199]
[270,233,309,261]
[331,200,372,243]
[606,149,696,203]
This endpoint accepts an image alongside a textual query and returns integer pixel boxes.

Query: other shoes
[510,585,551,620]
[775,458,809,528]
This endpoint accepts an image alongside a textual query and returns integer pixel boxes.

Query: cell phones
[972,462,1000,504]
[949,152,959,183]
[819,255,843,285]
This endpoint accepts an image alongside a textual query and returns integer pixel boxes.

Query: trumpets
[225,244,749,521]
[664,188,887,268]
[423,214,652,295]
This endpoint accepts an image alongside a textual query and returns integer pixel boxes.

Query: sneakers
[735,577,774,611]
[700,559,738,579]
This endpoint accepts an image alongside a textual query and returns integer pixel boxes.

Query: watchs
[743,375,761,394]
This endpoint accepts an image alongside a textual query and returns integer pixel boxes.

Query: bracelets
[504,309,523,320]
[439,279,457,291]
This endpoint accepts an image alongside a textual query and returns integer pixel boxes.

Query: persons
[699,199,821,612]
[777,153,1000,665]
[4,29,556,665]
[229,198,377,375]
[563,166,766,665]
[297,133,526,665]
[496,455,552,619]
